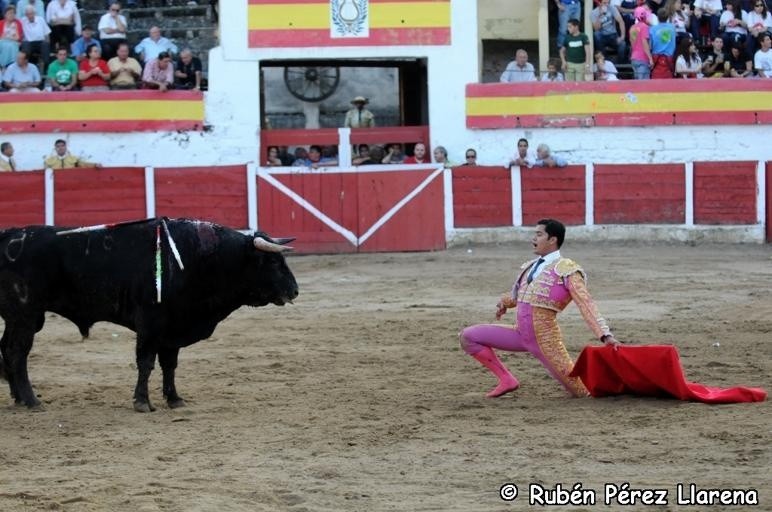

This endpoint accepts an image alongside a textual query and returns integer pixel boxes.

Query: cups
[725,62,729,70]
[717,54,724,63]
[707,56,713,63]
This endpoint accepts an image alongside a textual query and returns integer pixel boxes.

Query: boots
[470,346,520,397]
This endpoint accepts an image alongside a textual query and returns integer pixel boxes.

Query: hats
[634,6,652,18]
[81,23,91,31]
[350,96,369,103]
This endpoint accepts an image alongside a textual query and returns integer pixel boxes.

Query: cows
[0,216,299,414]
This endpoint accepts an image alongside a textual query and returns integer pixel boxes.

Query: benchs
[31,0,219,83]
[604,16,714,79]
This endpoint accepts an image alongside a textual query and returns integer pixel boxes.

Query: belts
[566,1,578,5]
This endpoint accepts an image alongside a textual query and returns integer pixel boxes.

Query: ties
[527,258,545,283]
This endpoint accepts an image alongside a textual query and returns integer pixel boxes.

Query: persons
[344,96,375,129]
[497,0,772,83]
[43,139,104,170]
[462,148,476,166]
[0,142,16,172]
[458,218,621,398]
[0,0,202,93]
[266,142,431,167]
[433,146,457,167]
[534,143,569,167]
[502,138,535,169]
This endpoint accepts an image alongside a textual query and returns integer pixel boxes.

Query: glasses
[466,156,476,158]
[755,3,764,7]
[111,8,120,13]
